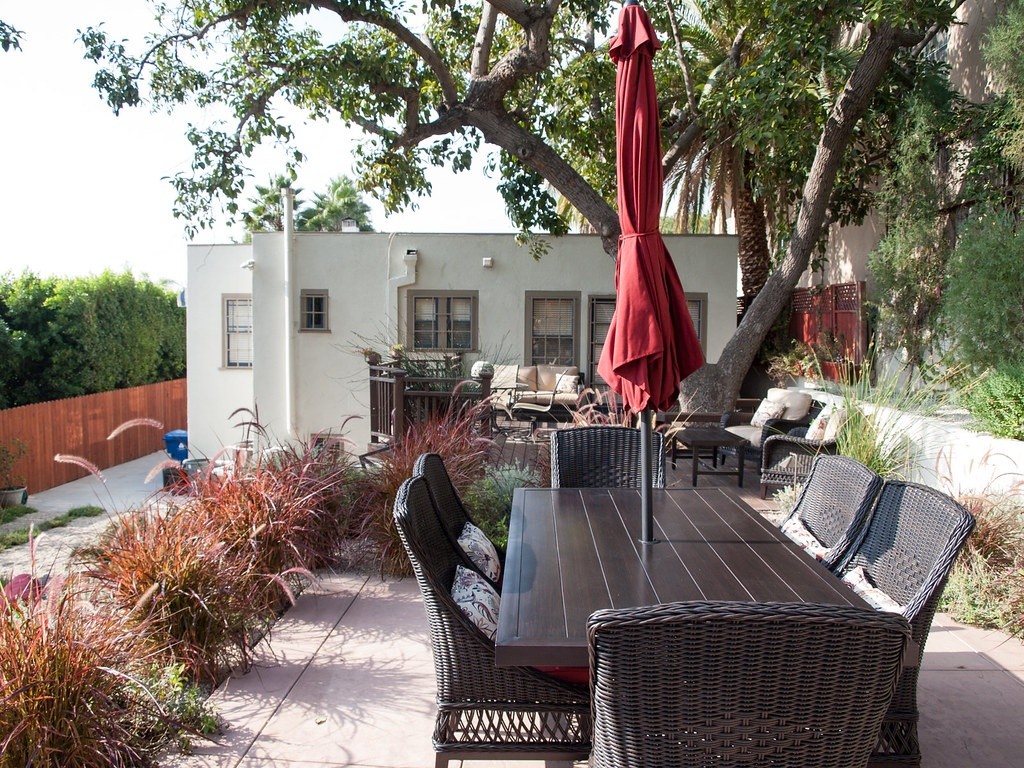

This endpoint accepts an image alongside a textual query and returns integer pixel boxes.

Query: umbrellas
[597,0,704,545]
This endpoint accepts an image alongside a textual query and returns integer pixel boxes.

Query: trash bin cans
[162,429,188,466]
[183,458,212,497]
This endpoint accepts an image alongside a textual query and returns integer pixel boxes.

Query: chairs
[412,452,507,591]
[391,474,592,768]
[586,600,915,768]
[550,426,665,490]
[832,480,977,768]
[759,417,854,499]
[513,370,568,442]
[719,401,828,475]
[778,452,884,571]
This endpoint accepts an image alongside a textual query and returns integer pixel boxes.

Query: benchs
[657,410,756,425]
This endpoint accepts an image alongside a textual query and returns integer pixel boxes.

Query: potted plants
[0,437,28,510]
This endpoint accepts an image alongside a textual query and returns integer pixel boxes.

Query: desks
[671,425,752,487]
[489,381,529,435]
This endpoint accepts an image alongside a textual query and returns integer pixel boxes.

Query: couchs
[491,369,587,421]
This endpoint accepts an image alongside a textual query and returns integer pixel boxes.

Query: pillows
[451,565,501,641]
[825,407,860,442]
[779,517,832,563]
[536,364,578,391]
[514,365,537,391]
[804,401,837,441]
[840,564,907,614]
[457,521,501,583]
[767,387,812,420]
[750,398,787,430]
[555,374,580,394]
